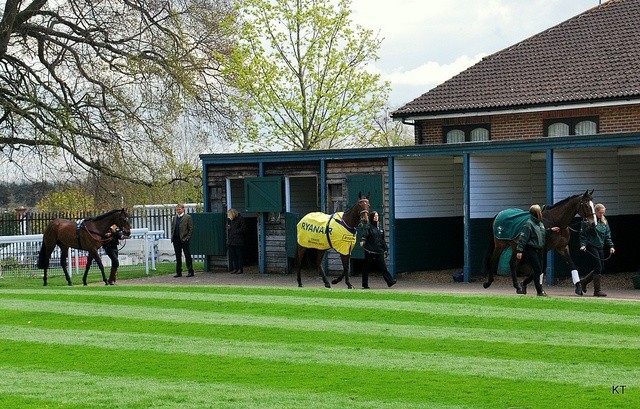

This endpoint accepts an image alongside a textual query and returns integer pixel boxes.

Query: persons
[359,210,397,289]
[171,204,195,277]
[102,224,126,285]
[226,209,244,274]
[579,203,615,297]
[517,204,560,296]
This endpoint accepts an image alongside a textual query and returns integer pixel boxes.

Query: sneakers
[520,281,526,294]
[537,290,547,296]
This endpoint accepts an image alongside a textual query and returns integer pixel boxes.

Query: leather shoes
[230,269,237,273]
[186,272,194,277]
[174,274,182,277]
[236,269,243,274]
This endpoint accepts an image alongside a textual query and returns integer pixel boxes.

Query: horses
[36,206,132,286]
[293,190,370,289]
[482,187,597,296]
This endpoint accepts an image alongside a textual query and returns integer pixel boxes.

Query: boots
[593,274,607,296]
[580,271,594,293]
